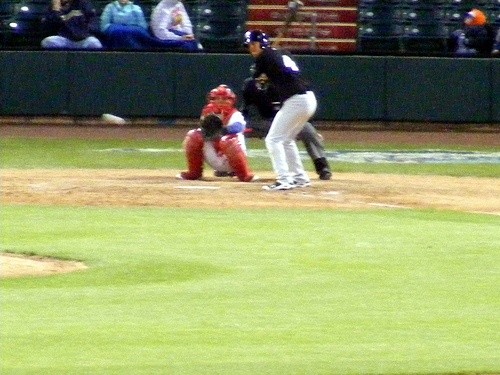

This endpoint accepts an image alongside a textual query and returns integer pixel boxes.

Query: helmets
[241,29,270,49]
[209,84,235,105]
[464,9,486,25]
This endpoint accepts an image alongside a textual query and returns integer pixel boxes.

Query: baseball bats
[272,1,300,48]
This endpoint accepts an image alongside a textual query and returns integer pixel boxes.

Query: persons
[178,85,256,183]
[452,9,491,58]
[41,0,104,51]
[150,0,203,52]
[100,0,149,51]
[240,30,332,191]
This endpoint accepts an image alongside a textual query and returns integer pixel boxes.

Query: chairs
[356,0,500,58]
[0,0,246,51]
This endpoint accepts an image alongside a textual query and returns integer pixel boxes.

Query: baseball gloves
[203,115,223,135]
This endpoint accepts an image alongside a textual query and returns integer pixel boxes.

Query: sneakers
[262,179,295,190]
[292,176,310,187]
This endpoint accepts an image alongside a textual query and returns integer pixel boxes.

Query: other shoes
[239,174,254,181]
[180,172,198,179]
[320,172,331,179]
